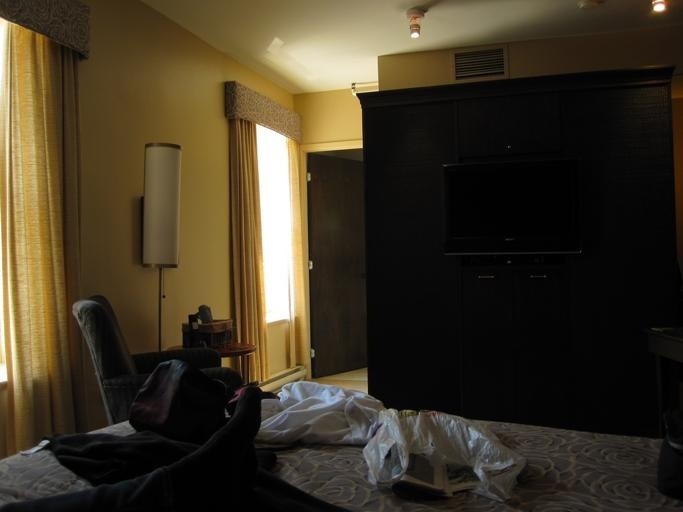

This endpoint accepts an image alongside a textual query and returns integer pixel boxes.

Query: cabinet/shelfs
[357,62,681,439]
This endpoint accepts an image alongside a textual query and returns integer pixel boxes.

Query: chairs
[72,295,222,425]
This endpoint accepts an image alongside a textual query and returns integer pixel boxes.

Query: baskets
[183,320,232,347]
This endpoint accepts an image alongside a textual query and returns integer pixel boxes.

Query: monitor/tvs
[440,152,584,257]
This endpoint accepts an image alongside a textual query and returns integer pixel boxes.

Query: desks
[646,326,682,438]
[166,342,257,386]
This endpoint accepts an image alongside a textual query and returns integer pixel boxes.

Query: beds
[3,396,683,512]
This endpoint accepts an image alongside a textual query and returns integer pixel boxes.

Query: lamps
[143,142,183,364]
[406,7,424,39]
